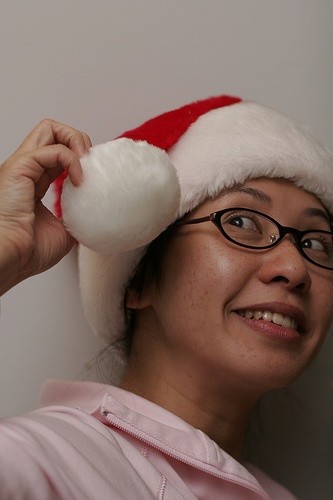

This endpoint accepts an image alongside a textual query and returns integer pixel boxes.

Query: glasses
[173,206,333,270]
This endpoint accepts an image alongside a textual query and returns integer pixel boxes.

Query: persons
[0,94,333,500]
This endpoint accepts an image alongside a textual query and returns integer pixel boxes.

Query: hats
[53,94,333,367]
